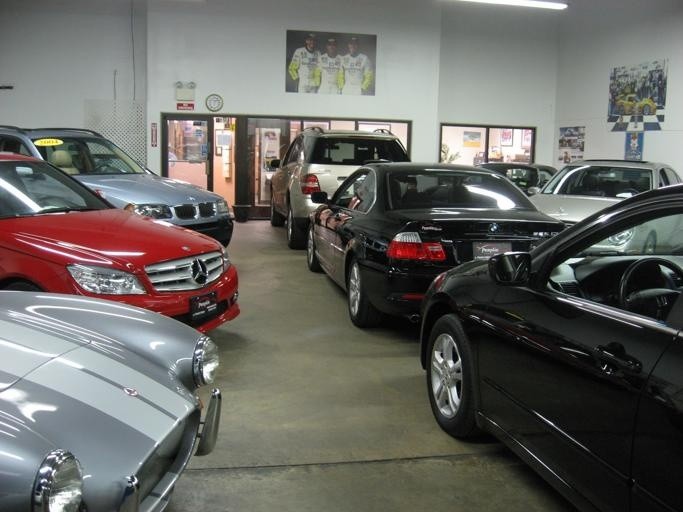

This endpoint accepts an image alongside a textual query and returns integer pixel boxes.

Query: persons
[288,33,374,95]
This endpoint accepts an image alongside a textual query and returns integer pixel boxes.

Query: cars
[523,160,680,256]
[560,341,615,379]
[466,162,558,198]
[614,94,656,116]
[306,160,567,329]
[188,158,201,164]
[168,152,177,167]
[338,226,367,245]
[0,152,242,333]
[418,181,682,511]
[458,300,559,360]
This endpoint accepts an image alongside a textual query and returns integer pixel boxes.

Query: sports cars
[0,289,224,512]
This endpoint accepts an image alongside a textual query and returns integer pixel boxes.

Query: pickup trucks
[472,152,503,167]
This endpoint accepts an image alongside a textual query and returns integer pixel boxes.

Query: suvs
[362,236,388,253]
[270,127,410,250]
[0,123,233,252]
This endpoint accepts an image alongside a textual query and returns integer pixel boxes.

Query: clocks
[205,93,223,111]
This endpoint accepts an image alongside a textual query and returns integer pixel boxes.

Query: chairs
[392,179,402,201]
[448,186,471,203]
[403,189,419,204]
[575,176,605,197]
[631,176,650,192]
[51,151,80,175]
